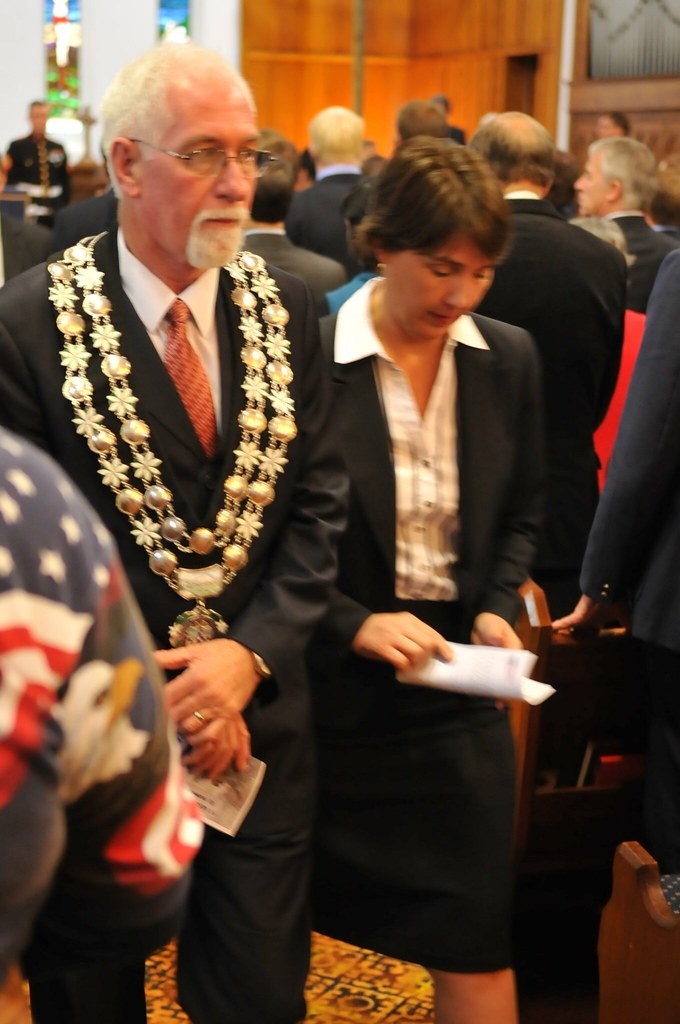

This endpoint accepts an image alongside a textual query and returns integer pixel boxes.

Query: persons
[0,419,206,1024]
[0,152,59,291]
[531,146,582,229]
[434,109,632,730]
[588,111,628,134]
[353,132,392,186]
[245,133,559,1024]
[636,150,680,264]
[550,207,653,514]
[44,136,120,252]
[240,90,481,314]
[0,39,353,1023]
[520,243,680,1024]
[0,96,81,224]
[564,132,668,316]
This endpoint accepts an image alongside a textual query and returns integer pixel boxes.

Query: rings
[193,710,209,725]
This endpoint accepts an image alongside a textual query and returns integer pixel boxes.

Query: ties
[163,298,220,455]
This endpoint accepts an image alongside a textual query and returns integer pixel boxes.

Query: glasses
[127,137,277,180]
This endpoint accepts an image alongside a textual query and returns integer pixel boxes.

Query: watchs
[246,649,271,679]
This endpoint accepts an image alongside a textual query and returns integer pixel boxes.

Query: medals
[46,228,298,663]
